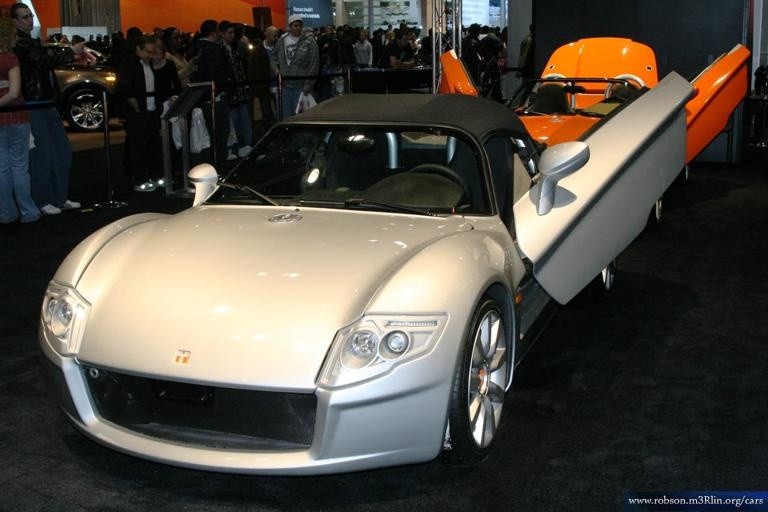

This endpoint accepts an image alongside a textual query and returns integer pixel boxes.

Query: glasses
[21,13,33,19]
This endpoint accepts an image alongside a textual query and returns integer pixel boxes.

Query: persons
[506,23,532,108]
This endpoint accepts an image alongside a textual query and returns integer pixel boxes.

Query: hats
[288,14,303,25]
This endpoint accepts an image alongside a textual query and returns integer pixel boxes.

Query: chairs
[524,83,640,116]
[317,129,511,191]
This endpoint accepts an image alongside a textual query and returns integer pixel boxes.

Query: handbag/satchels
[169,108,211,153]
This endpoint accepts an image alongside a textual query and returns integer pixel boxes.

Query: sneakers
[238,145,254,158]
[62,200,81,209]
[39,204,62,215]
[134,179,159,192]
[227,149,238,161]
